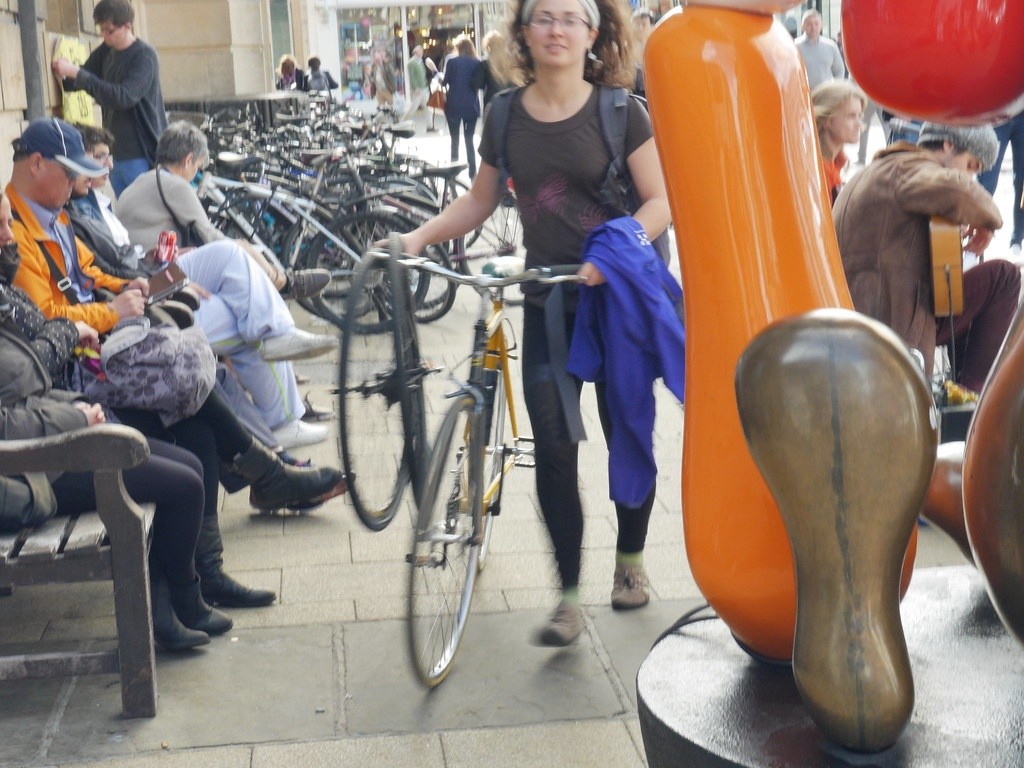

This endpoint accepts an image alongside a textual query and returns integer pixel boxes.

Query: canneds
[153,229,177,264]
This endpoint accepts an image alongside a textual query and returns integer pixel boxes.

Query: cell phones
[146,263,189,304]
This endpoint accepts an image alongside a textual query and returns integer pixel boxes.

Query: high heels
[151,575,211,651]
[165,564,233,634]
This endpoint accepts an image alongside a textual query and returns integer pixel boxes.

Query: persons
[0,191,233,652]
[881,108,923,145]
[977,110,1024,259]
[474,31,525,111]
[832,120,1021,396]
[373,0,673,645]
[811,79,868,210]
[62,124,339,448]
[794,8,845,91]
[69,121,196,279]
[114,119,331,301]
[4,116,340,511]
[425,34,486,178]
[272,54,338,102]
[400,44,430,121]
[375,50,395,110]
[52,0,168,211]
[852,98,892,169]
[631,7,654,98]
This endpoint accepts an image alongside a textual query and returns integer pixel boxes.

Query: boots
[234,434,342,508]
[194,511,277,607]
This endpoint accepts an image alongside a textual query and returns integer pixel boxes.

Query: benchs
[0,426,159,718]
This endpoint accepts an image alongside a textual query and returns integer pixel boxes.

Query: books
[146,263,189,304]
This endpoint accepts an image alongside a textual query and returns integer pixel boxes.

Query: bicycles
[331,235,593,681]
[189,101,530,334]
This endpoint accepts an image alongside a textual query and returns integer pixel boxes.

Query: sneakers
[541,600,587,644]
[611,562,649,611]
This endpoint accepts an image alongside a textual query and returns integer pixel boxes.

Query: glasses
[528,14,593,29]
[94,152,114,164]
[100,23,124,34]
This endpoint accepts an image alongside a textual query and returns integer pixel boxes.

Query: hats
[11,115,104,178]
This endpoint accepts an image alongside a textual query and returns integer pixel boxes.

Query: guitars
[929,214,966,318]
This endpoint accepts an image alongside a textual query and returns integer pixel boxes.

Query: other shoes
[271,417,330,449]
[1009,243,1024,266]
[426,127,438,134]
[277,453,357,512]
[259,326,339,362]
[300,390,333,422]
[295,372,311,384]
[279,268,332,300]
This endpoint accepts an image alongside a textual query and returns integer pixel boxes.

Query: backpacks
[307,69,332,101]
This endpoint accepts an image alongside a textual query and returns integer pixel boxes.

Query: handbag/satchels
[83,316,217,429]
[427,80,447,110]
